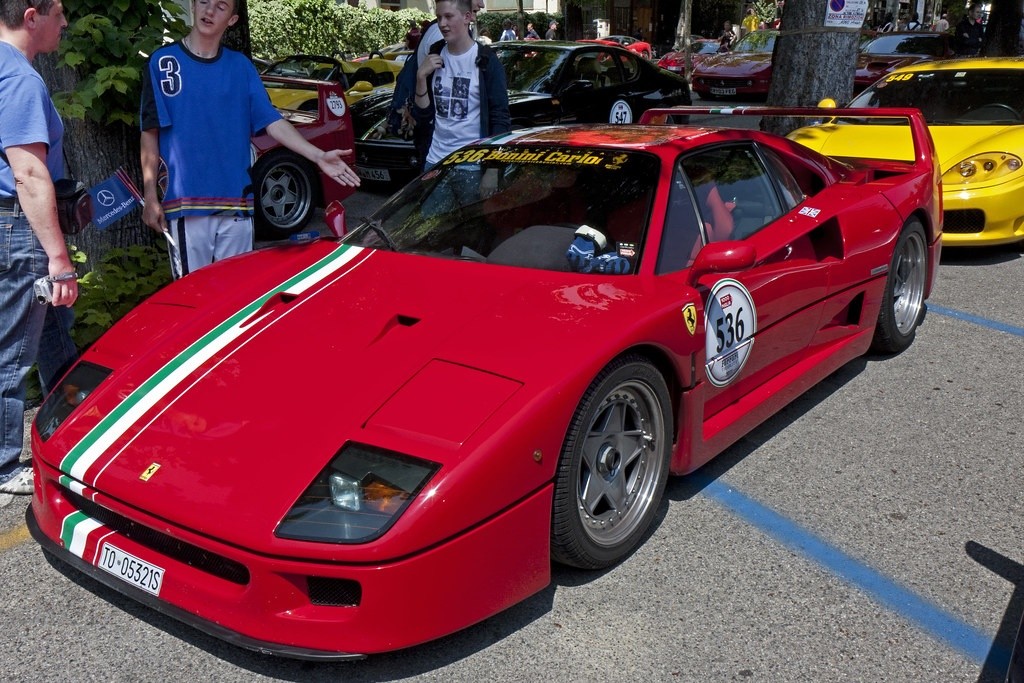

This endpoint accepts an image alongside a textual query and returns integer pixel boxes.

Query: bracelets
[415,90,428,97]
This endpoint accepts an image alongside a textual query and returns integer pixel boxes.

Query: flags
[88,167,140,229]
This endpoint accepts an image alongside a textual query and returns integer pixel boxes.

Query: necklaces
[184,37,189,49]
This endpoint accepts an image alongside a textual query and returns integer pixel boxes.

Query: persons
[897,3,985,57]
[718,9,760,53]
[140,0,361,280]
[410,0,560,219]
[0,0,81,495]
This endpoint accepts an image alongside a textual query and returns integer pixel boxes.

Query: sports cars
[852,30,965,99]
[689,27,782,104]
[656,37,722,82]
[347,38,695,192]
[781,54,1023,259]
[575,33,653,64]
[248,38,418,241]
[26,93,946,665]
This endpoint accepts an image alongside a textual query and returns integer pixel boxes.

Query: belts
[0,195,22,208]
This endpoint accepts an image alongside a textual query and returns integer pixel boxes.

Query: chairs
[606,67,630,86]
[575,56,602,87]
[307,67,349,91]
[476,164,583,255]
[349,66,382,90]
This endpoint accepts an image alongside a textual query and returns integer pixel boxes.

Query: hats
[550,20,560,25]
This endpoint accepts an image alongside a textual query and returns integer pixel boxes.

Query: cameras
[34,275,54,305]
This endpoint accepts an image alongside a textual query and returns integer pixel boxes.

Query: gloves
[566,224,608,269]
[582,252,637,275]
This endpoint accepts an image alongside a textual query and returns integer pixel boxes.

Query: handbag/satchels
[51,178,93,234]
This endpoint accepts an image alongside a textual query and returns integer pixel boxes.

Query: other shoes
[0,464,35,494]
